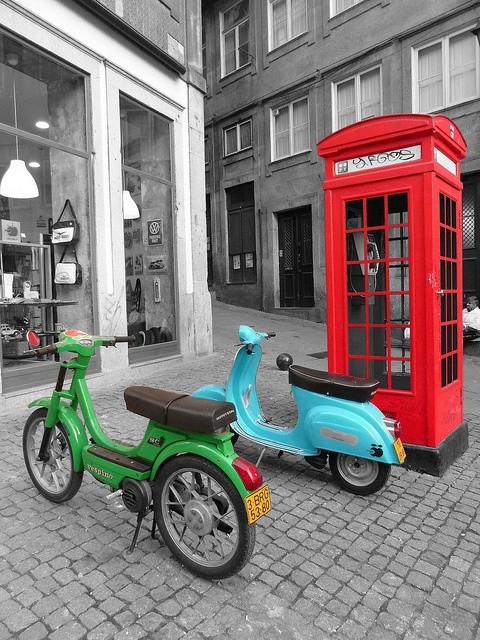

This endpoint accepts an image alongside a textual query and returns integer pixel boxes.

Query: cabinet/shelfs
[0,242,77,364]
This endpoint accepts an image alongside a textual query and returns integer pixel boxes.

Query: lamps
[120,96,140,220]
[0,53,40,200]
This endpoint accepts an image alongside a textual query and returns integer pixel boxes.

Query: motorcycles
[14,328,273,581]
[189,323,406,495]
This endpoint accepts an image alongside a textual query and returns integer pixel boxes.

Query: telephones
[348,232,380,275]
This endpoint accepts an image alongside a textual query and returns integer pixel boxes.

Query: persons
[462,294,480,343]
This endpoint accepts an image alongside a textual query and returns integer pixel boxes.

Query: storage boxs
[3,342,29,359]
